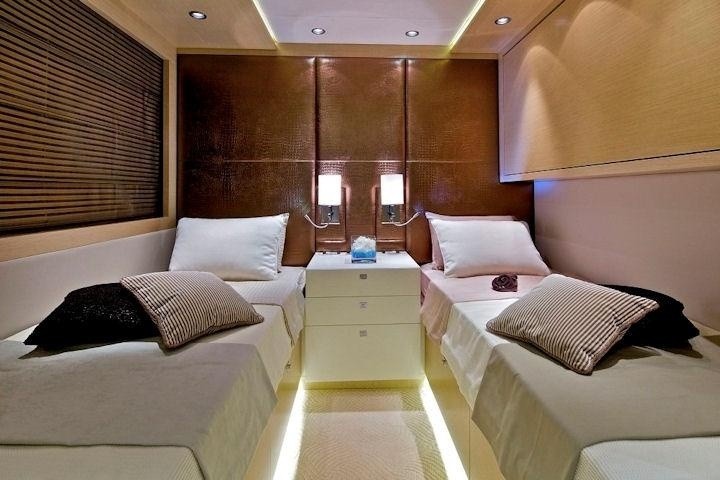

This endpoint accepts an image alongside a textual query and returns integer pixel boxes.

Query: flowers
[492,272,518,293]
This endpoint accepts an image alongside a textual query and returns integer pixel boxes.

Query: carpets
[296,382,448,480]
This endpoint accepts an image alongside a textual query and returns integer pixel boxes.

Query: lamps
[303,174,347,244]
[374,173,422,241]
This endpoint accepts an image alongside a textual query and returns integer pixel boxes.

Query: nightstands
[306,249,422,390]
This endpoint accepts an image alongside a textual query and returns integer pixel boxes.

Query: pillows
[429,218,553,277]
[119,270,264,349]
[597,279,699,361]
[424,210,518,270]
[22,281,157,348]
[168,213,290,281]
[486,272,658,374]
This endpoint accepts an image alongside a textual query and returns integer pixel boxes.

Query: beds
[0,211,306,479]
[418,209,719,479]
